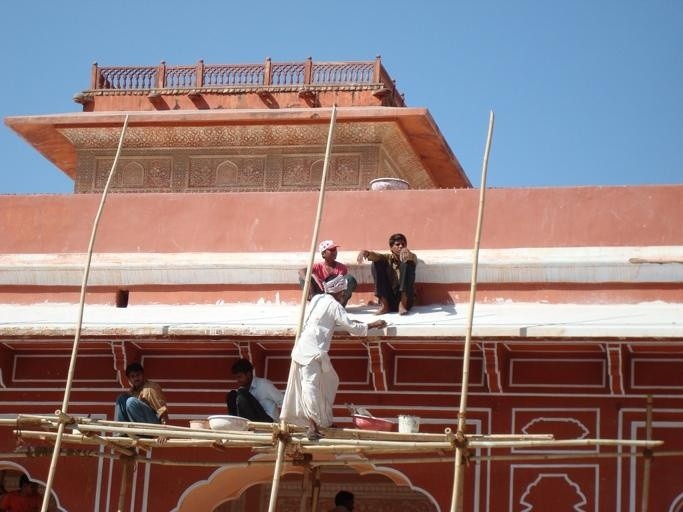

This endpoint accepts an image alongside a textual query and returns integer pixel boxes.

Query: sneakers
[307,430,327,441]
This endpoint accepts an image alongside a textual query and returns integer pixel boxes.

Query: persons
[280,274,387,440]
[299,239,358,311]
[353,231,418,316]
[224,359,284,423]
[113,363,170,445]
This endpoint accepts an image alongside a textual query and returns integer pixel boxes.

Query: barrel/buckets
[396,413,422,435]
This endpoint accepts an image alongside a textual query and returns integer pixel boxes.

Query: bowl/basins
[396,414,420,433]
[349,412,398,431]
[206,414,251,432]
[188,420,211,433]
[367,176,410,192]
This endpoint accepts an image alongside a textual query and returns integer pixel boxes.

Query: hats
[319,240,341,254]
